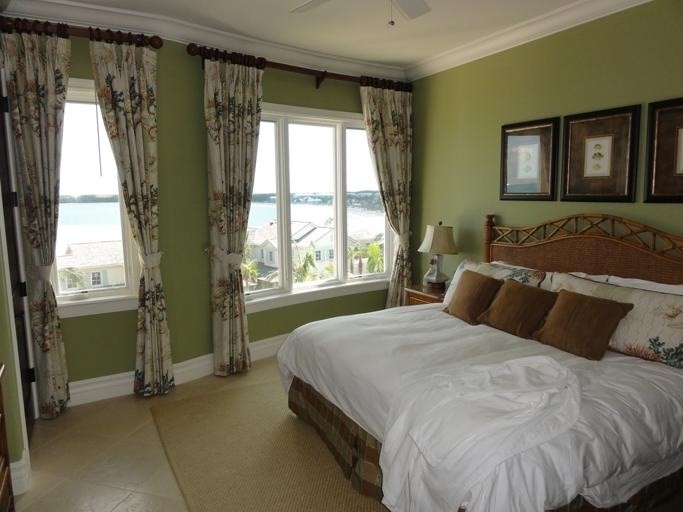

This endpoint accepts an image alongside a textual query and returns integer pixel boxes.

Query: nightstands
[403,282,452,305]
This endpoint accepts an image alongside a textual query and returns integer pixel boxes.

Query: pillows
[437,256,682,369]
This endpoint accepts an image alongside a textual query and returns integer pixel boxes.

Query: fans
[286,0,433,25]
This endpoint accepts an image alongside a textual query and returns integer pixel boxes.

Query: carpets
[147,374,392,511]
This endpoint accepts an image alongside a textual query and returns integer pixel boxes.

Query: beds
[269,209,683,512]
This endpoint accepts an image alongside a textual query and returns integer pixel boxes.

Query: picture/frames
[497,115,560,203]
[640,94,682,207]
[558,102,644,204]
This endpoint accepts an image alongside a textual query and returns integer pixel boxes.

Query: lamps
[415,220,460,284]
